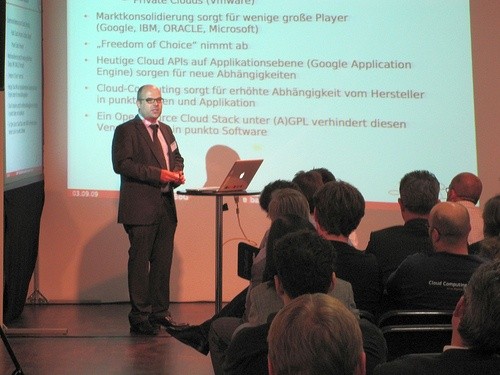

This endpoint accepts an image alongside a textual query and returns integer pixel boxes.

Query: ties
[148,124,164,159]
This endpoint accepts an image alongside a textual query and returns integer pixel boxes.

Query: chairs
[377,309,453,361]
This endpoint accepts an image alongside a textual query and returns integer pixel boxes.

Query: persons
[468,193,500,259]
[222,230,388,375]
[381,204,485,312]
[111,85,185,335]
[367,170,441,279]
[267,294,368,375]
[376,260,500,375]
[445,172,486,243]
[165,168,369,357]
[208,188,312,374]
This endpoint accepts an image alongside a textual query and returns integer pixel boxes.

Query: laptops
[186,160,264,193]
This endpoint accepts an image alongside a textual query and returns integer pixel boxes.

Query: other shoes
[130,326,158,336]
[165,325,209,356]
[154,314,172,327]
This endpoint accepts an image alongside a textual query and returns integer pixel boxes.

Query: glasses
[137,97,163,104]
[445,187,450,192]
